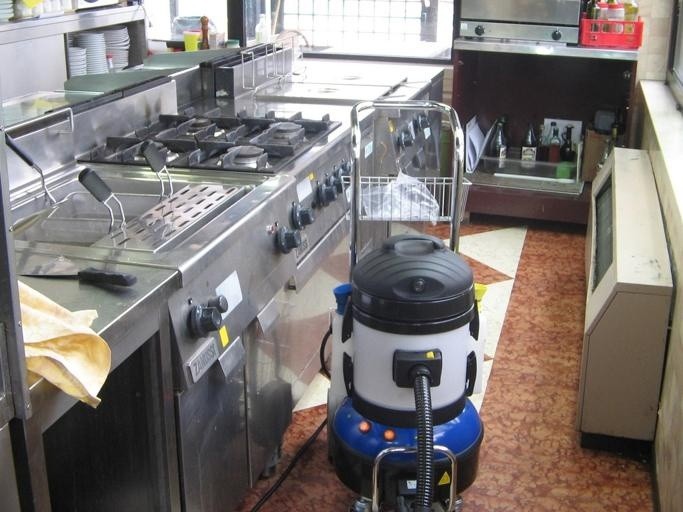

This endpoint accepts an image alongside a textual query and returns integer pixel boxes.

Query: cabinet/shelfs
[447,52,638,226]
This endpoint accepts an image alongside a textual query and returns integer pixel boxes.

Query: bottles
[254,13,268,44]
[587,1,639,34]
[488,120,625,176]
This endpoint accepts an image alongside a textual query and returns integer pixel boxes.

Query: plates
[65,23,131,78]
[0,0,15,24]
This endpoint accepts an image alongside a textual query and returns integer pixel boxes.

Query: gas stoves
[74,106,352,266]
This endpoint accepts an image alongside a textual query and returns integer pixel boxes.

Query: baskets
[580,11,643,49]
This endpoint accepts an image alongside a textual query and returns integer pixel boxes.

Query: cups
[184,31,198,53]
[14,0,64,22]
